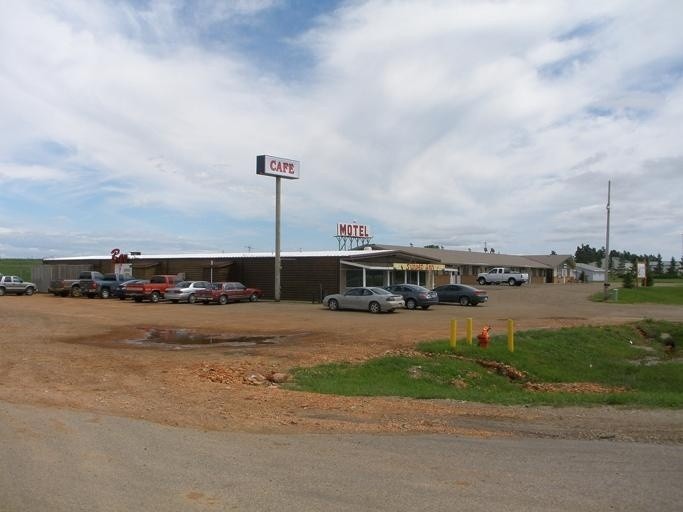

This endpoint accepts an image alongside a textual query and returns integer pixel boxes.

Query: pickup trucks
[475,267,528,286]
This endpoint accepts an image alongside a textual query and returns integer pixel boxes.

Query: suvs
[0,275,36,296]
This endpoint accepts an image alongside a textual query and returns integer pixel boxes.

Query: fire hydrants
[476,326,490,349]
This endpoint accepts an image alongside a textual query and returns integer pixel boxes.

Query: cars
[384,284,438,311]
[429,283,488,306]
[321,287,406,313]
[47,270,264,304]
